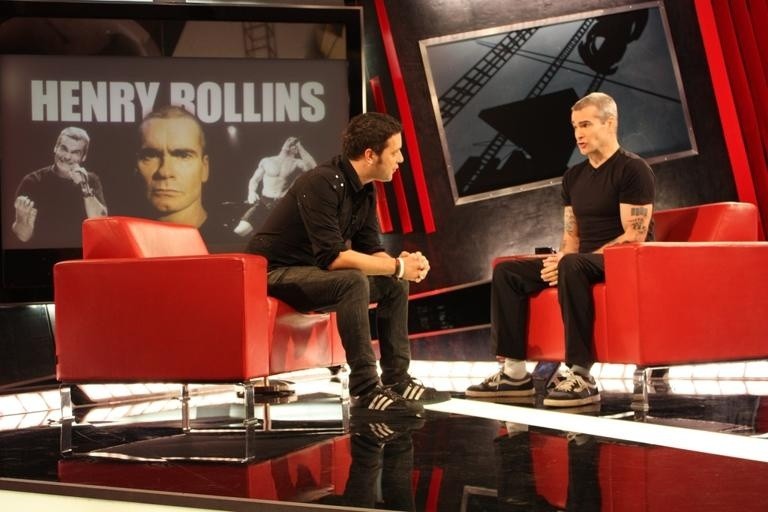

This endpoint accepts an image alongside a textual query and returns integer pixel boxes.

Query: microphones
[70,161,87,198]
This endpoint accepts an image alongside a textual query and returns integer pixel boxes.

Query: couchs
[54,214,350,464]
[492,202,768,420]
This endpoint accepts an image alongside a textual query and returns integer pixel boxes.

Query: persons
[246,112,451,416]
[465,93,654,409]
[12,127,107,247]
[311,416,426,511]
[233,136,316,237]
[129,107,244,245]
[492,421,605,511]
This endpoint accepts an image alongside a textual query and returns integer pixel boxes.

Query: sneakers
[386,379,452,404]
[543,376,602,406]
[349,387,425,416]
[544,404,601,441]
[351,418,426,444]
[465,397,536,422]
[464,372,535,395]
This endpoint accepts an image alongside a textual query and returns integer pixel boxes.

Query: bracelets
[397,257,405,281]
[393,258,400,278]
